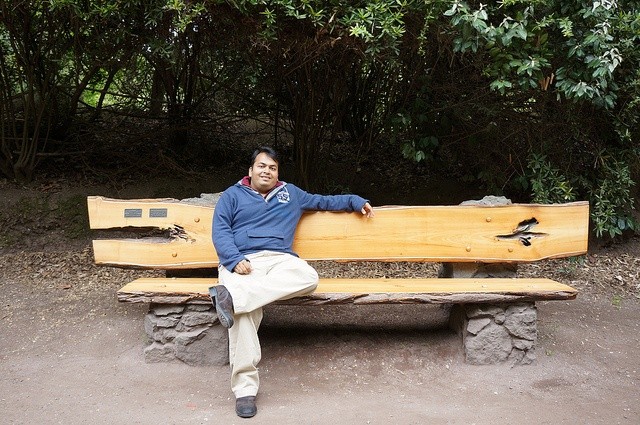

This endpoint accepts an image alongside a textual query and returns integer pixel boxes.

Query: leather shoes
[235,396,257,418]
[209,285,233,328]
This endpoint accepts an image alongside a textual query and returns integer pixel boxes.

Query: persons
[209,147,373,419]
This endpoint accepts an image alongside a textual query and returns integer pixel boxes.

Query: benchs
[85,190,590,367]
